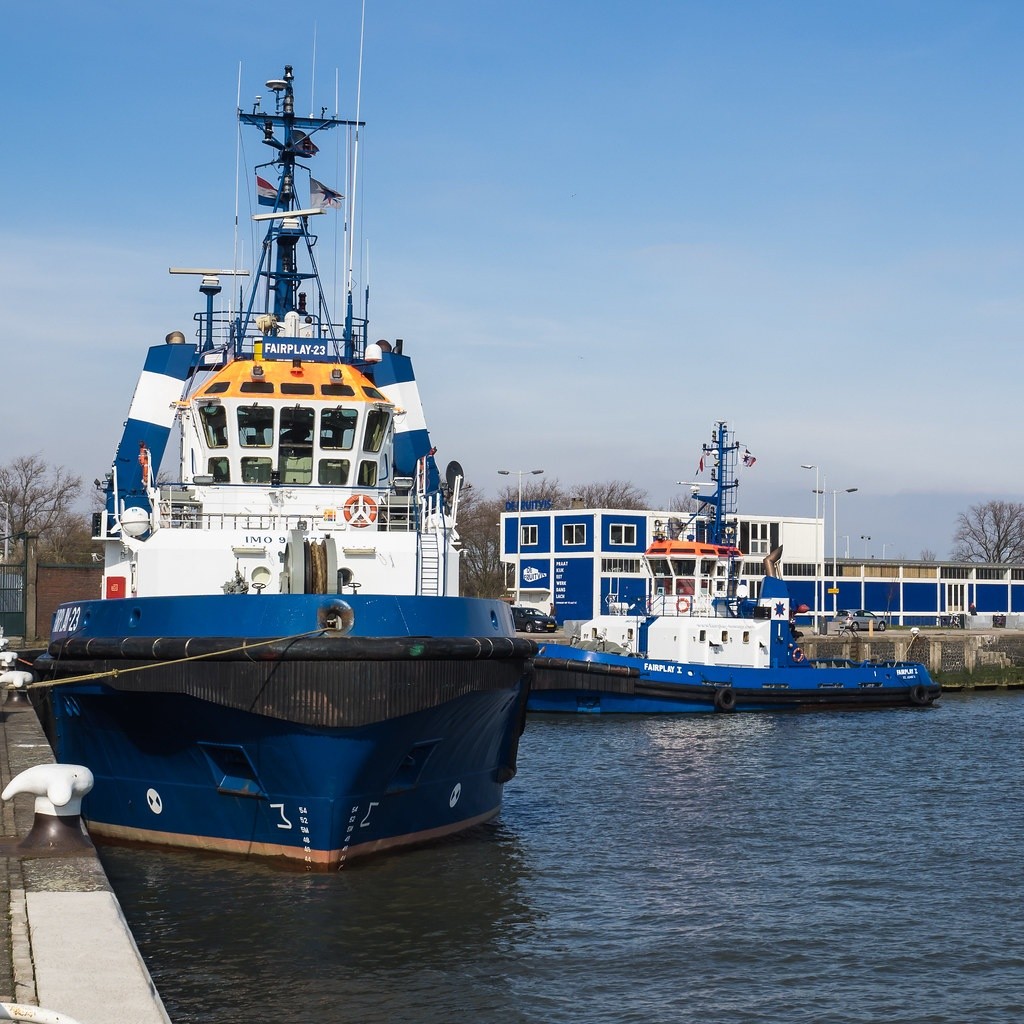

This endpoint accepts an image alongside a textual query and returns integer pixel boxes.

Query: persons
[549,603,555,618]
[970,603,977,615]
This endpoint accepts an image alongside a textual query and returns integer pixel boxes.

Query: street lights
[800,464,821,634]
[811,487,860,619]
[497,468,545,604]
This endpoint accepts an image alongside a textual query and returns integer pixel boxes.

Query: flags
[257,176,285,208]
[695,458,703,475]
[741,449,756,467]
[310,178,345,209]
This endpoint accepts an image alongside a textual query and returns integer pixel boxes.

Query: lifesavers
[715,688,736,712]
[343,494,378,529]
[675,598,689,613]
[910,683,943,706]
[136,444,151,485]
[793,648,804,663]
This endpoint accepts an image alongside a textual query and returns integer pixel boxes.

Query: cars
[833,608,888,632]
[510,605,558,634]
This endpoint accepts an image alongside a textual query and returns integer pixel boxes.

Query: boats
[1,1,537,870]
[525,418,946,713]
